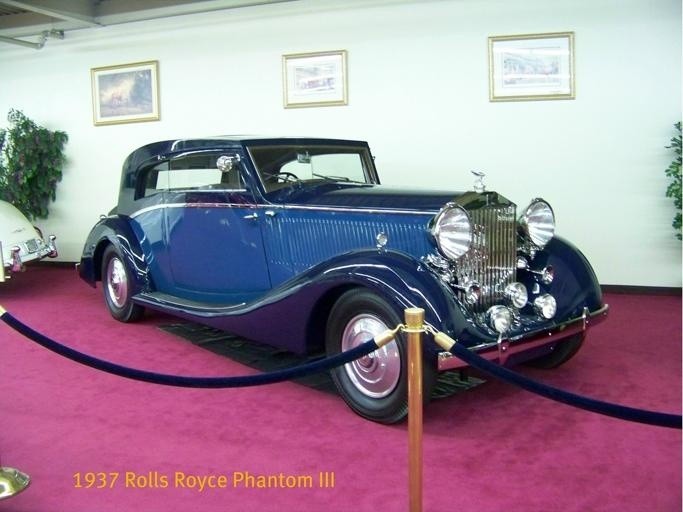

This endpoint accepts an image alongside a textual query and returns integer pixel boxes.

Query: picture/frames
[280,49,349,108]
[485,31,576,102]
[90,60,160,126]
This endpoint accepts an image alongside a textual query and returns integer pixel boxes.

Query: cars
[79,129,612,427]
[0,195,57,285]
